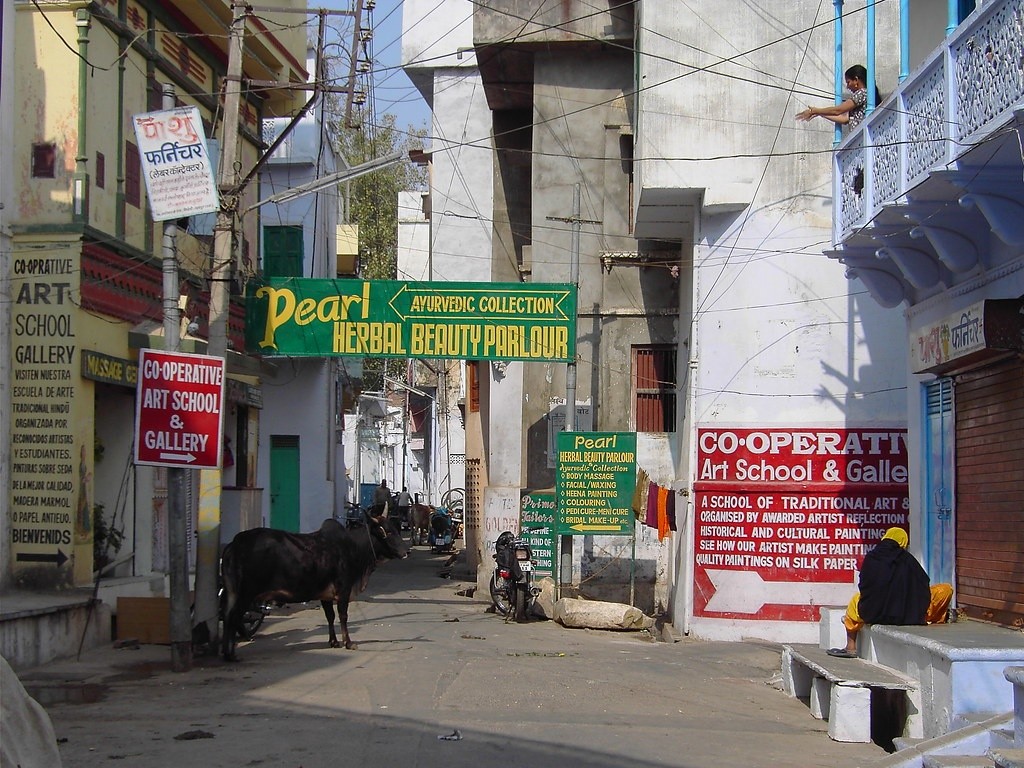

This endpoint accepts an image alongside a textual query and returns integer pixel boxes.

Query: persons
[826,527,954,658]
[397,487,414,516]
[794,64,881,134]
[372,479,393,517]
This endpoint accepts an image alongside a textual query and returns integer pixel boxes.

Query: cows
[219,499,412,664]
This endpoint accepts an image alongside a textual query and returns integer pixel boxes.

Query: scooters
[345,501,363,529]
[429,512,452,552]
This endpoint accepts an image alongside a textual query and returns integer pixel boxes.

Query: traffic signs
[134,347,226,469]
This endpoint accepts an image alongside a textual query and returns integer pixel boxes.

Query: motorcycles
[490,525,544,623]
[191,558,270,639]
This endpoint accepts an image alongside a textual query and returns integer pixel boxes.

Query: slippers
[827,648,857,659]
